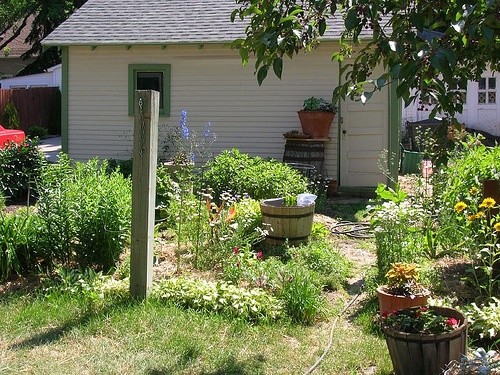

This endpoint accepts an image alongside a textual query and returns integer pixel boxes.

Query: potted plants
[378,261,431,317]
[298,96,338,139]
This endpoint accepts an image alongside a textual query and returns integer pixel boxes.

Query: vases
[164,162,195,183]
[260,199,316,248]
[382,306,469,375]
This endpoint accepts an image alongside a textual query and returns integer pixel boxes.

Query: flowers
[368,305,458,340]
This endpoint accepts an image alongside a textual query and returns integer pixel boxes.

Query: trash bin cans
[401,150,424,175]
